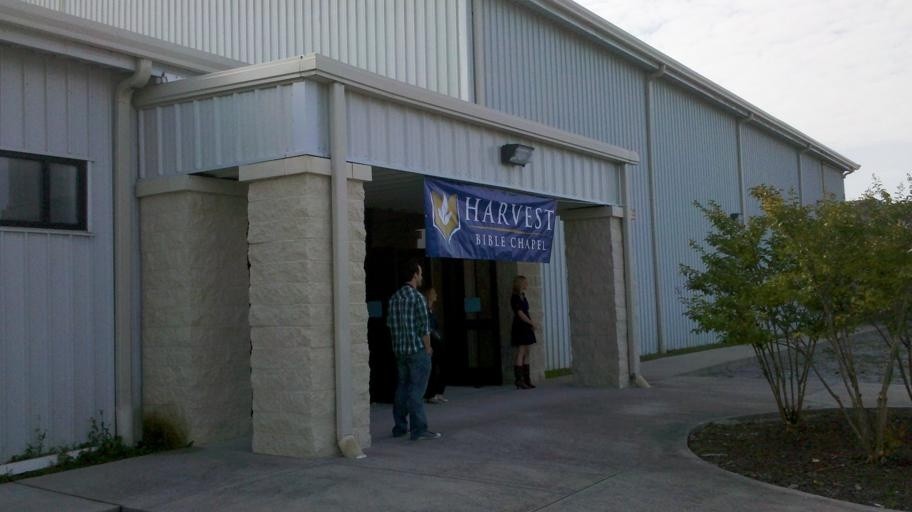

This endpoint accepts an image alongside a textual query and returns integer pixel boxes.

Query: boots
[514,364,536,390]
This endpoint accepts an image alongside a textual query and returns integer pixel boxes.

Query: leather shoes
[428,395,448,404]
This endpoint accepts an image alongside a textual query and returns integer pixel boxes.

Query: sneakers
[392,430,441,440]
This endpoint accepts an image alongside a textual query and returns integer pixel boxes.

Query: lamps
[501,143,535,167]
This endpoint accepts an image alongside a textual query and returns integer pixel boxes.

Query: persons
[386,262,441,443]
[419,282,449,405]
[507,275,540,390]
